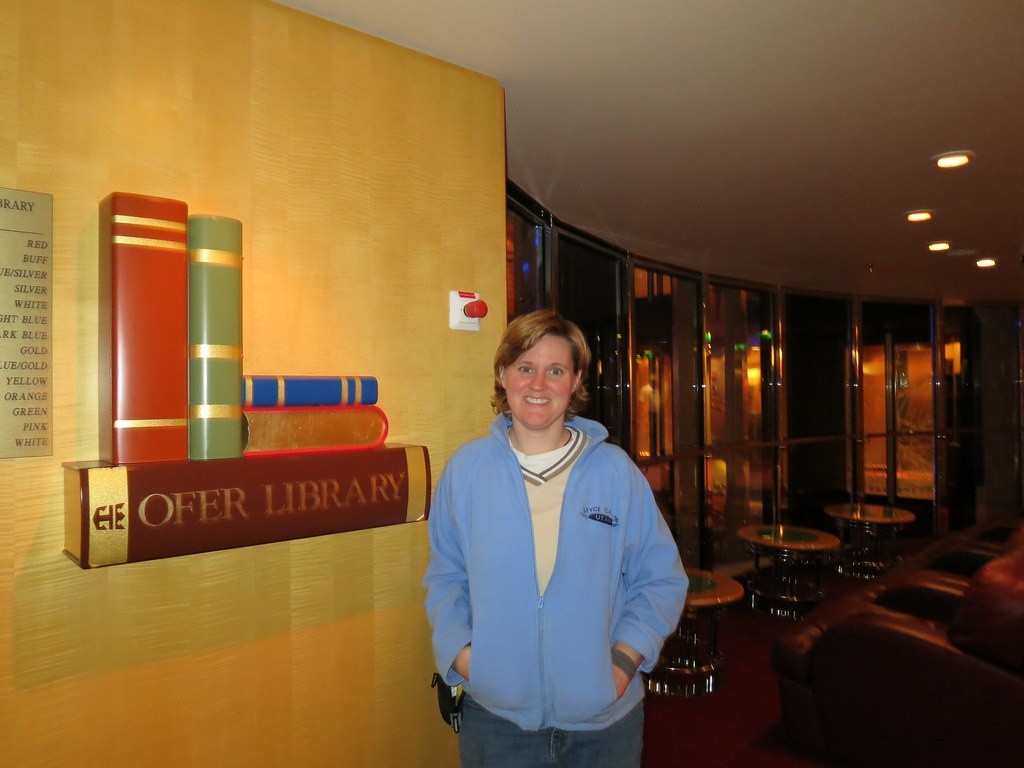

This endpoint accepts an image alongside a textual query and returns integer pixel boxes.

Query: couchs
[773,521,1023,767]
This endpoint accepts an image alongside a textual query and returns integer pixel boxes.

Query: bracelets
[611,645,638,680]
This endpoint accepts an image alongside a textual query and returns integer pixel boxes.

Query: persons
[421,308,691,768]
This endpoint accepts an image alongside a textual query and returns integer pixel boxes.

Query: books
[61,190,433,573]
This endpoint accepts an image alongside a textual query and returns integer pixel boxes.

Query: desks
[738,524,840,602]
[649,568,745,676]
[825,503,917,571]
